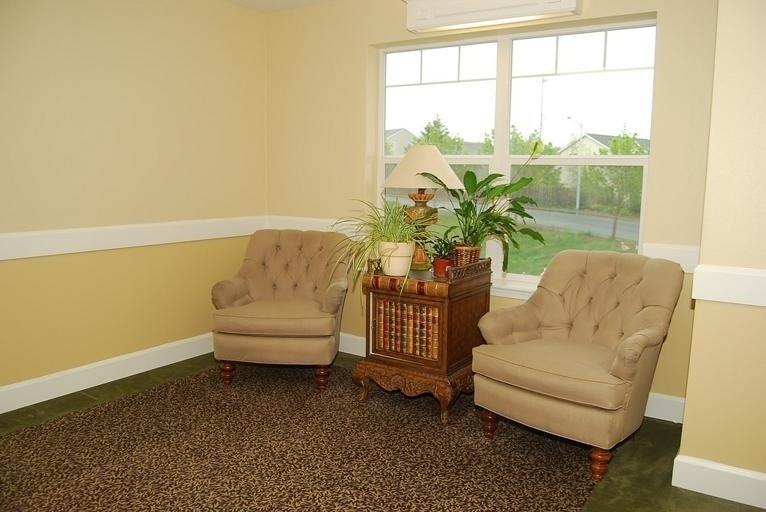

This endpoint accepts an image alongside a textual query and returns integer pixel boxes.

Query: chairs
[471,249,683,483]
[211,229,351,393]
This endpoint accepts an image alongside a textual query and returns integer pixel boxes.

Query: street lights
[535,77,551,137]
[567,115,585,211]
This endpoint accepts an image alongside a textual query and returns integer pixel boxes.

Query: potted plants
[326,140,545,315]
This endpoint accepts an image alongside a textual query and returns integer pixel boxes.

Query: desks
[352,257,493,426]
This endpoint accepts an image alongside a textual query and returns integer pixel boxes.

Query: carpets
[0,363,617,512]
